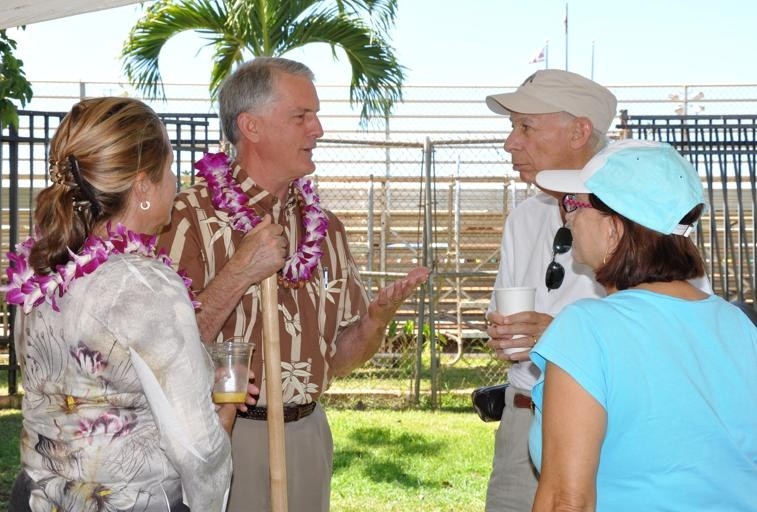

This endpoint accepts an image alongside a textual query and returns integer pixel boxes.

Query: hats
[485,69,617,136]
[535,139,708,238]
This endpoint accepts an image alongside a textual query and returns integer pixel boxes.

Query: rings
[529,334,537,349]
[390,295,401,305]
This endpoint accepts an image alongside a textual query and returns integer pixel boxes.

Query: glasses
[545,227,573,292]
[560,194,597,214]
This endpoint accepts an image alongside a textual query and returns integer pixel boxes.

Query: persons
[150,56,431,512]
[482,68,713,511]
[6,94,260,512]
[529,137,756,511]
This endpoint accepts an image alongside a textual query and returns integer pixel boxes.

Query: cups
[492,285,539,356]
[205,340,257,404]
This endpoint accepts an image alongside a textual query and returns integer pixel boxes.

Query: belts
[235,402,317,423]
